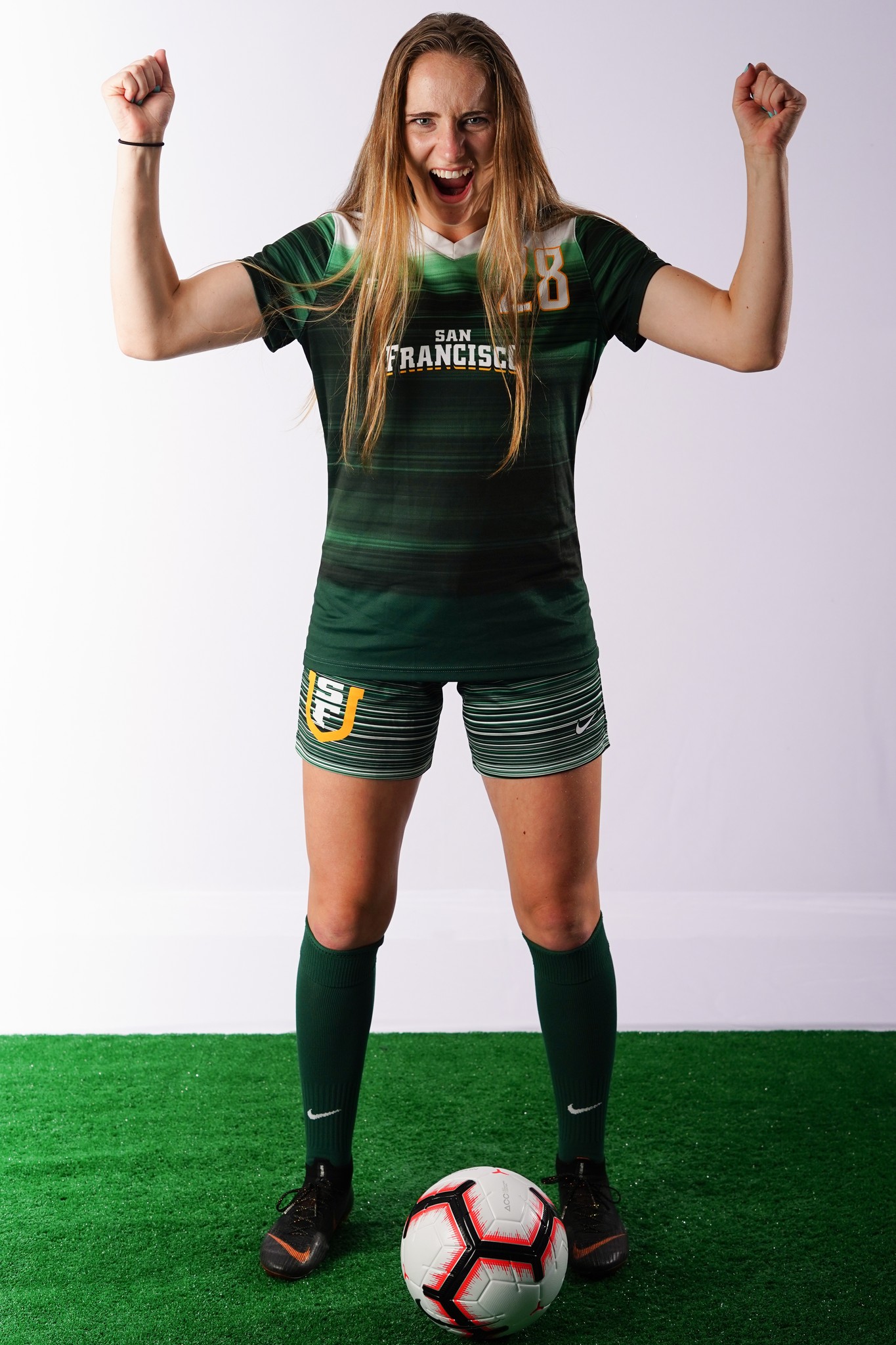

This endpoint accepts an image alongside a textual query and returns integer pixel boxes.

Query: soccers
[399,1166,571,1340]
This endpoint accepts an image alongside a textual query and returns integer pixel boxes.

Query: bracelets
[116,137,165,148]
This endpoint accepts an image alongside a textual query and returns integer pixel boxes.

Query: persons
[104,11,810,1287]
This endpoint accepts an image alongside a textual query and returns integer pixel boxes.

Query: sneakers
[261,1158,354,1280]
[542,1153,628,1280]
